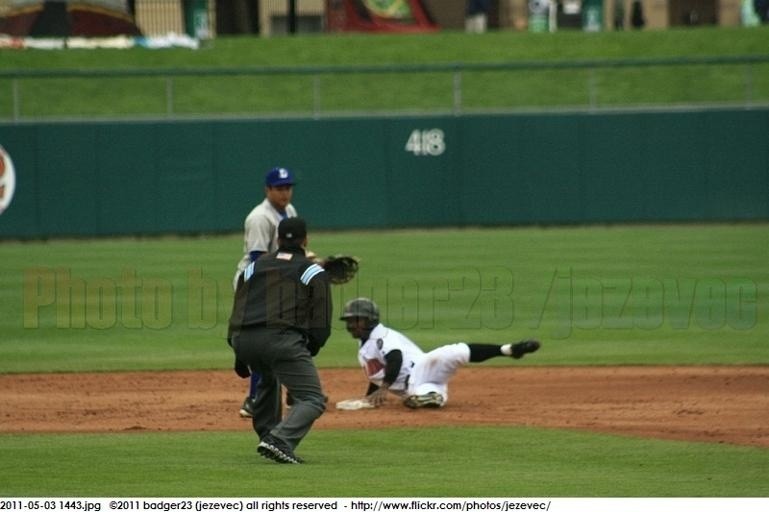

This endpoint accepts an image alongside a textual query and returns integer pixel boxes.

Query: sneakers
[240,397,255,418]
[404,390,443,408]
[257,434,302,463]
[286,390,328,409]
[511,340,539,358]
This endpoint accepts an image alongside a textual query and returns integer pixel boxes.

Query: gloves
[308,342,320,356]
[235,359,252,377]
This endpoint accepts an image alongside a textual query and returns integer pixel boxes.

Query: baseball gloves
[324,255,359,285]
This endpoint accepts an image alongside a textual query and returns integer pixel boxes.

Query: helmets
[341,298,380,321]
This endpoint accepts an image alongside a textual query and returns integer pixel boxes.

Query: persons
[233,163,358,416]
[230,215,334,465]
[339,294,543,410]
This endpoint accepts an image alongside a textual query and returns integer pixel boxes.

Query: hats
[265,167,294,188]
[278,216,306,240]
